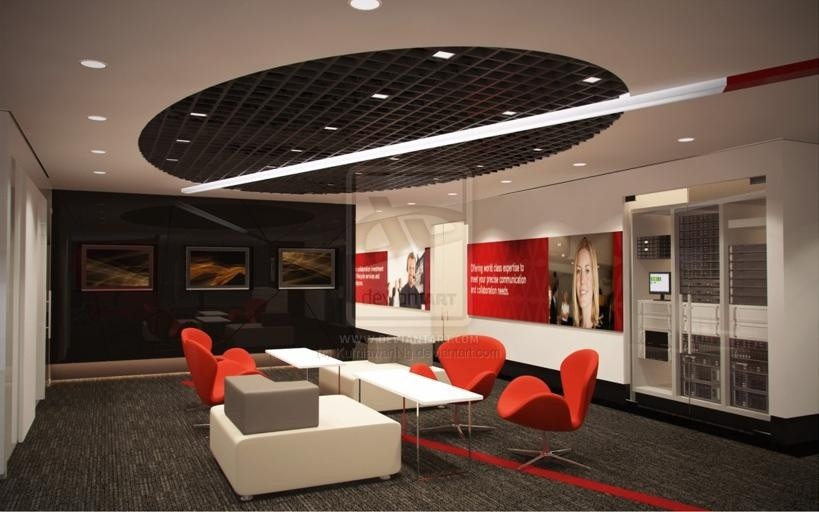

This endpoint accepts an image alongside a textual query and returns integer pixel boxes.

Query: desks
[264,346,346,381]
[350,369,486,479]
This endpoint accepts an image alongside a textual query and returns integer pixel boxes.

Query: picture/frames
[278,247,337,294]
[183,244,250,294]
[80,243,156,293]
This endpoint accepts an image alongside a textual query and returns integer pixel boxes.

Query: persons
[560,291,570,325]
[398,252,422,310]
[572,235,601,330]
[387,277,402,306]
[549,286,558,325]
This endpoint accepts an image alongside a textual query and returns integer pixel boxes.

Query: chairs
[410,335,507,445]
[497,348,600,472]
[178,326,257,413]
[183,337,268,430]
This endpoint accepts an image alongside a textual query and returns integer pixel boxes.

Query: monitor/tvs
[648,272,671,301]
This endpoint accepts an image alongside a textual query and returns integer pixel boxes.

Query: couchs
[207,372,403,502]
[318,336,454,413]
